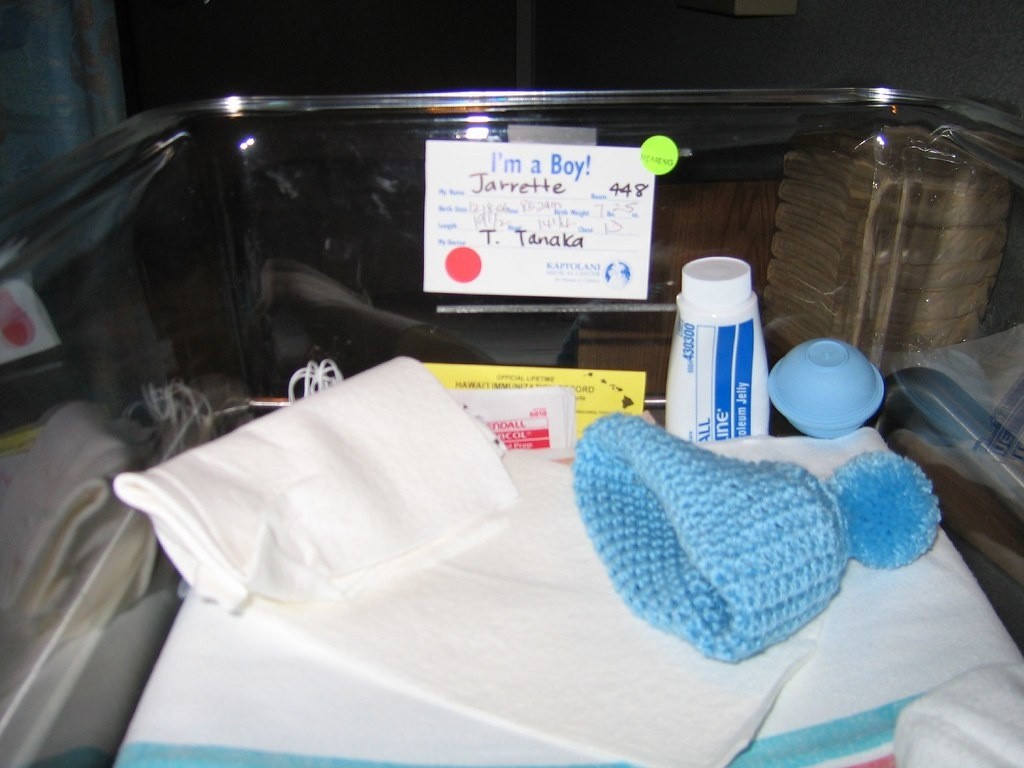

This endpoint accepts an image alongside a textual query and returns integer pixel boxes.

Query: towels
[893,658,1024,768]
[111,353,818,768]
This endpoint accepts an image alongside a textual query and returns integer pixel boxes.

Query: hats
[573,412,942,665]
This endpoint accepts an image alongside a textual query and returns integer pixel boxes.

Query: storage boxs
[0,84,1024,768]
